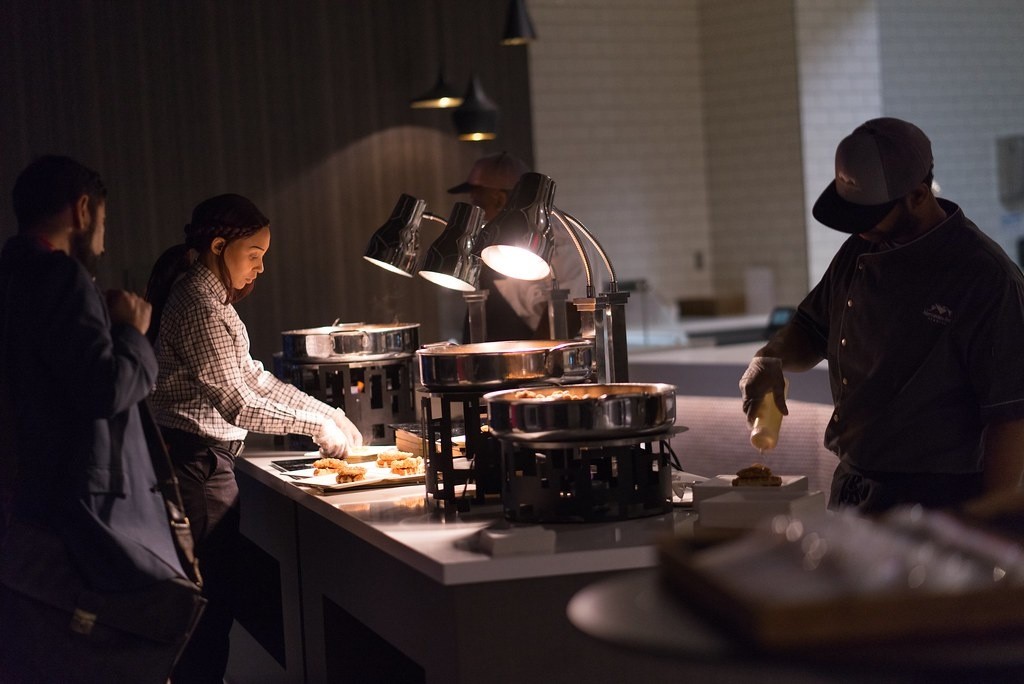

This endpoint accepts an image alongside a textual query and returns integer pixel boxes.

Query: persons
[0,149,190,684]
[141,190,366,683]
[443,147,616,343]
[736,117,1024,553]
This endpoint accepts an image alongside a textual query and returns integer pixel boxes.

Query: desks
[234,443,840,684]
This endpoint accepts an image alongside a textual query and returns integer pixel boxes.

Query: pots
[484,382,677,438]
[281,322,420,363]
[416,341,592,389]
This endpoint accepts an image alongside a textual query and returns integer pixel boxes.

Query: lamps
[478,173,632,382]
[419,202,601,339]
[364,193,489,344]
[410,0,540,143]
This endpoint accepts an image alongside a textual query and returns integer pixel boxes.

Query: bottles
[750,377,789,455]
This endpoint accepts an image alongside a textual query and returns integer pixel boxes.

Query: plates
[280,458,425,489]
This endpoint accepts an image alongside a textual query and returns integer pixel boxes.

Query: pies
[736,463,772,477]
[391,458,417,474]
[732,477,782,486]
[313,458,346,475]
[336,467,365,482]
[377,452,407,467]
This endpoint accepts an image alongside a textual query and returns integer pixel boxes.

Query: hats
[812,118,933,233]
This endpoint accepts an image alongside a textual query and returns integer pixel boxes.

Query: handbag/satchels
[0,516,209,684]
